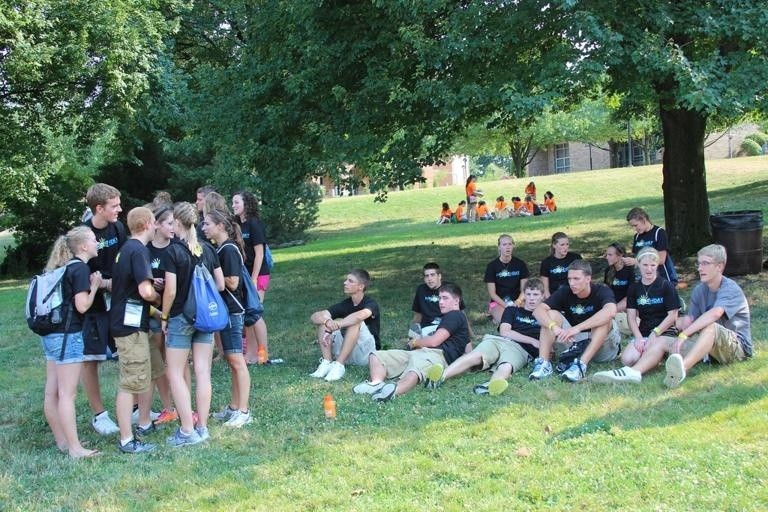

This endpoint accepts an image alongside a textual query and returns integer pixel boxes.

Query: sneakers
[61,405,253,460]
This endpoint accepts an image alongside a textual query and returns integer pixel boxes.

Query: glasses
[694,261,719,266]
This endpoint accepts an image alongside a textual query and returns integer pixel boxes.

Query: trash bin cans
[709,210,763,277]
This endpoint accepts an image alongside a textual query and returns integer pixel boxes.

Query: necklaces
[642,280,654,300]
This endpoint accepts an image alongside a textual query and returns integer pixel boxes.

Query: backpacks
[636,227,678,286]
[175,240,231,333]
[217,244,264,327]
[266,245,272,270]
[26,259,82,361]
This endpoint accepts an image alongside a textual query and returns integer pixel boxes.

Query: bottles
[503,296,514,306]
[323,395,336,418]
[257,347,267,364]
[50,292,63,323]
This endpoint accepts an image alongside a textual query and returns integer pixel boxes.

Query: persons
[603,241,642,336]
[407,263,482,344]
[538,232,583,300]
[484,234,527,326]
[436,174,557,225]
[528,258,621,382]
[619,245,682,367]
[422,279,546,395]
[310,269,381,382]
[38,182,268,457]
[620,206,679,288]
[354,284,471,402]
[592,243,753,387]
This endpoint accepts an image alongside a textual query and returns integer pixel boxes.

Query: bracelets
[653,326,662,336]
[548,321,557,331]
[324,319,330,326]
[678,332,688,340]
[325,330,332,334]
